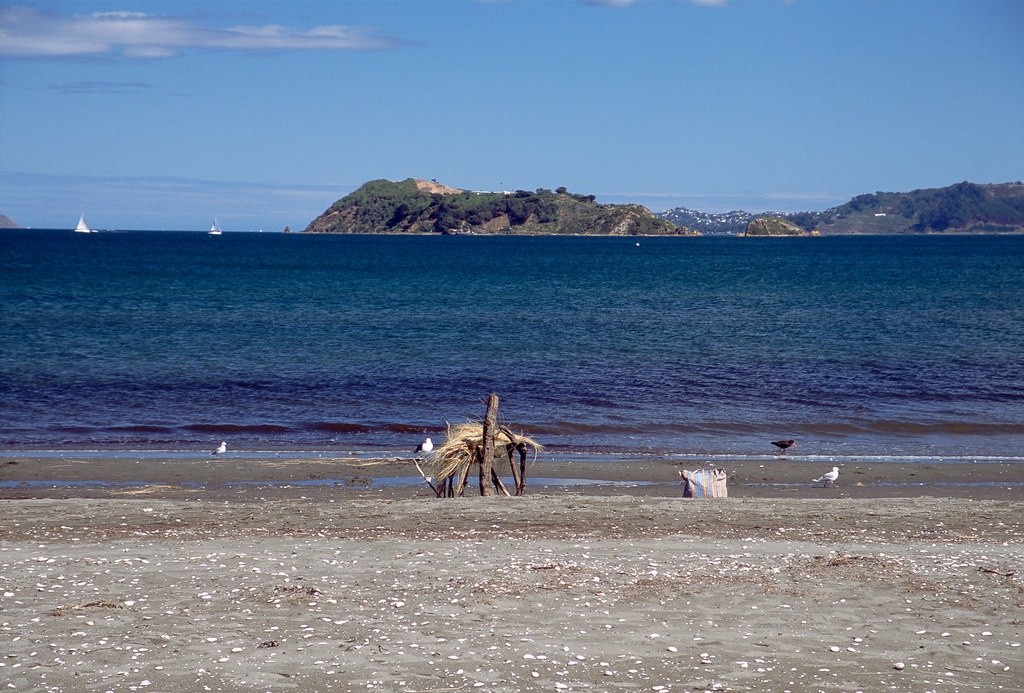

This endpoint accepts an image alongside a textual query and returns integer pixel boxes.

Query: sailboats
[74,215,90,233]
[208,217,221,235]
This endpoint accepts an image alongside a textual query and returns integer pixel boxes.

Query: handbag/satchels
[681,461,728,499]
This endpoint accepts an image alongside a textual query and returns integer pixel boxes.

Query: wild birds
[212,442,227,454]
[421,438,434,453]
[771,439,797,452]
[816,466,841,488]
